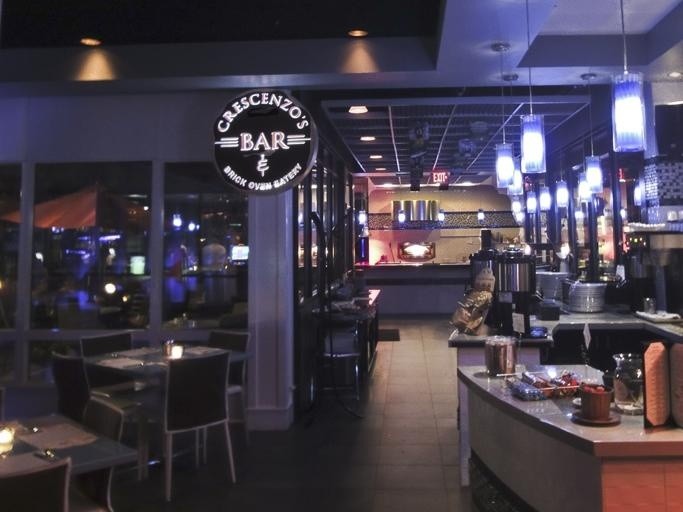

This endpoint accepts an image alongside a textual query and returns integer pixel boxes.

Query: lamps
[477,186,485,222]
[358,194,367,225]
[73,20,113,50]
[436,209,446,223]
[609,0,644,154]
[496,1,603,223]
[398,209,406,222]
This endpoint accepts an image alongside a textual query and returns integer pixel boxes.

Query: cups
[583,383,613,418]
[643,298,655,313]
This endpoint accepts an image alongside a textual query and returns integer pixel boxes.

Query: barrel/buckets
[485,335,517,376]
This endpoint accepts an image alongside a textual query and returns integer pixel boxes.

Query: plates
[536,272,607,313]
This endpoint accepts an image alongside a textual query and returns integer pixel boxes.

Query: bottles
[612,353,645,414]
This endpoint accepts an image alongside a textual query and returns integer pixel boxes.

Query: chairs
[138,349,236,502]
[1,457,71,511]
[79,396,124,510]
[78,330,150,412]
[51,350,149,480]
[208,328,252,445]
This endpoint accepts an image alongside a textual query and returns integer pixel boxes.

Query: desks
[1,413,139,512]
[82,346,248,479]
